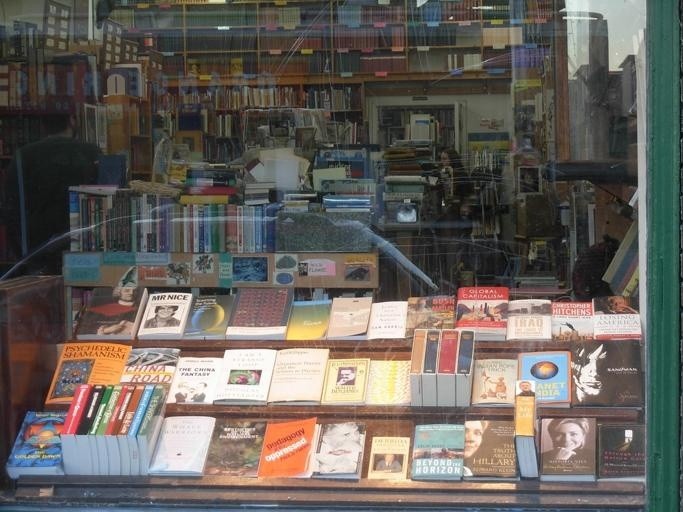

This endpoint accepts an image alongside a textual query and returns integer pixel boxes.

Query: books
[150,28,185,53]
[407,23,458,48]
[410,394,541,483]
[446,46,482,74]
[470,145,501,275]
[441,127,456,148]
[45,341,331,406]
[0,1,149,153]
[333,50,408,74]
[185,27,258,52]
[334,25,406,49]
[365,328,644,409]
[260,50,333,76]
[148,5,184,28]
[149,52,186,82]
[499,234,573,298]
[1,274,68,448]
[482,46,546,73]
[481,21,555,47]
[136,292,193,340]
[184,2,258,27]
[75,286,149,340]
[441,108,456,127]
[597,423,646,483]
[302,84,361,111]
[259,24,333,52]
[333,0,406,24]
[150,82,299,114]
[186,52,259,81]
[481,0,554,22]
[540,417,597,483]
[406,0,482,24]
[367,436,411,482]
[258,0,332,26]
[322,357,370,406]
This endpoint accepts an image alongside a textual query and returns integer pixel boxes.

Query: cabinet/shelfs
[333,0,407,75]
[0,55,105,162]
[258,0,332,75]
[63,183,380,339]
[184,0,258,75]
[106,94,152,181]
[407,0,482,76]
[152,82,365,171]
[15,308,647,511]
[380,205,445,300]
[110,5,184,75]
[482,0,552,76]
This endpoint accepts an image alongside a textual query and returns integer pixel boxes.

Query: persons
[543,417,591,466]
[571,233,633,313]
[440,148,477,272]
[335,366,357,386]
[373,453,403,473]
[0,109,108,276]
[77,286,140,335]
[520,168,540,193]
[541,417,594,475]
[143,304,181,329]
